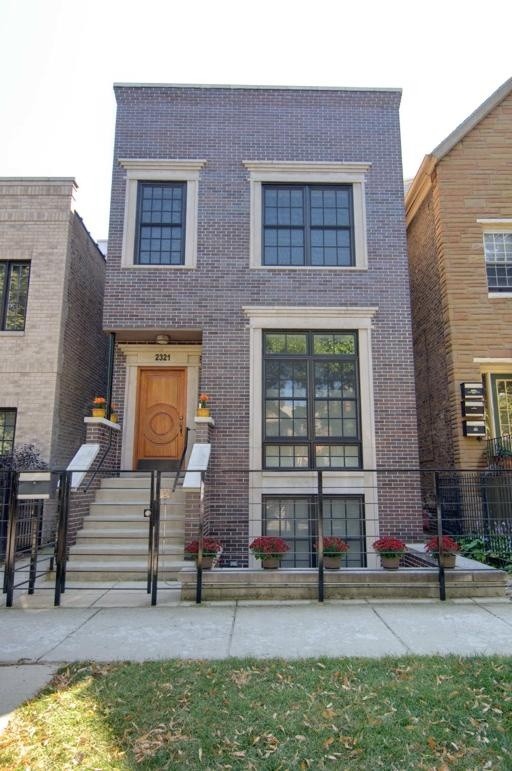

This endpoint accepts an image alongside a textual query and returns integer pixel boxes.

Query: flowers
[182,534,461,558]
[92,396,120,411]
[198,392,209,408]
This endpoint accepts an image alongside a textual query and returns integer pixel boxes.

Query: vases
[194,552,457,569]
[92,408,120,423]
[196,408,210,419]
